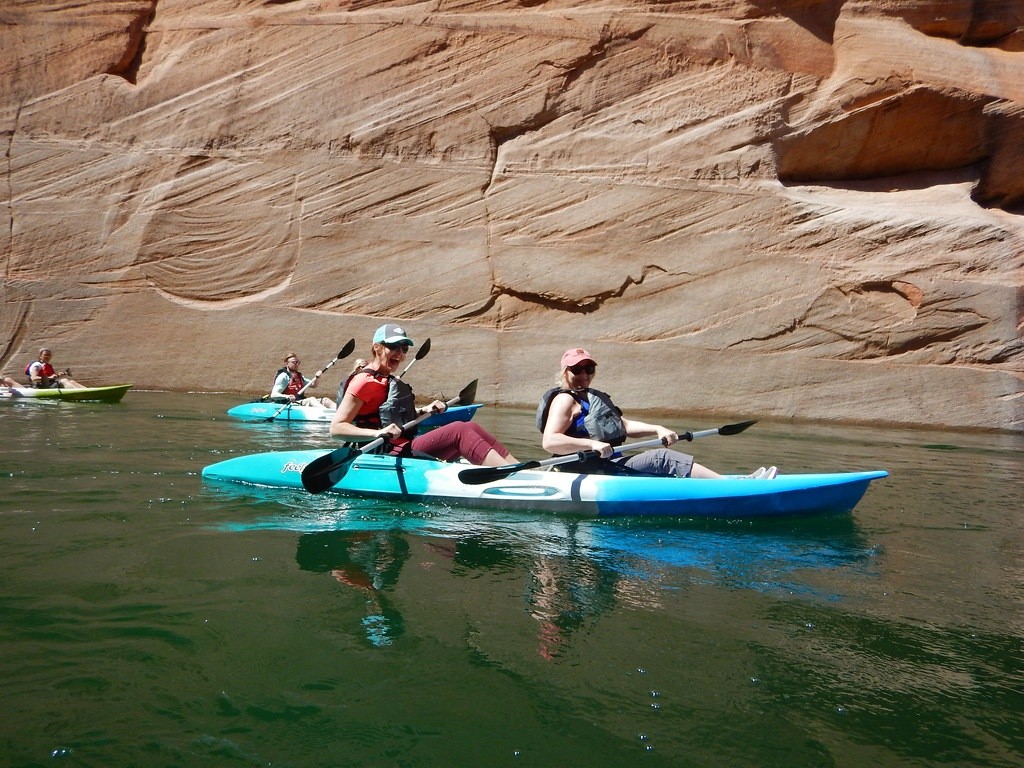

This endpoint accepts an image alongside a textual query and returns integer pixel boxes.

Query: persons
[0,375,24,388]
[329,324,520,466]
[354,358,368,371]
[25,348,87,389]
[271,353,337,409]
[536,350,778,480]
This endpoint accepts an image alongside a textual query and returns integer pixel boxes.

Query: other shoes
[525,463,560,472]
[757,466,778,480]
[738,466,766,479]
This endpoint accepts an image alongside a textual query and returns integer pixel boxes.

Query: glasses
[380,342,408,354]
[287,361,301,364]
[567,364,595,375]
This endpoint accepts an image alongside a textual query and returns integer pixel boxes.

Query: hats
[561,348,598,374]
[373,324,413,346]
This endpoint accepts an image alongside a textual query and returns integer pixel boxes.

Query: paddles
[263,337,357,421]
[301,378,478,495]
[40,367,73,388]
[457,417,762,485]
[395,338,433,381]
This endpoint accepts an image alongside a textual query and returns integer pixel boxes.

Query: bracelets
[419,407,424,415]
